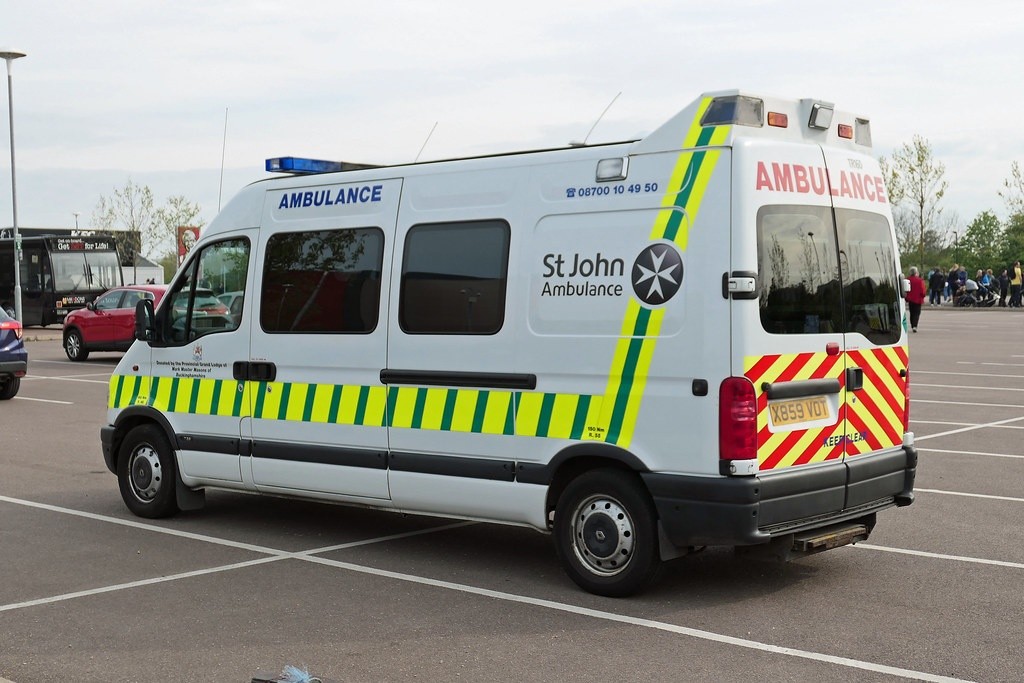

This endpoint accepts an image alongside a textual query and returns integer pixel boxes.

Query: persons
[905,261,1024,333]
[146,278,225,297]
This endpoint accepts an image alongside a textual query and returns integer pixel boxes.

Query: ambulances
[100,87,918,601]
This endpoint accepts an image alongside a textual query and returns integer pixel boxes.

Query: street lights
[0,47,28,335]
[952,230,958,263]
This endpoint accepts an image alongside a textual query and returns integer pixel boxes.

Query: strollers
[954,279,979,307]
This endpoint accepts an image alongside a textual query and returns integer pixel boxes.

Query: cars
[218,290,245,318]
[0,308,29,400]
[61,284,233,362]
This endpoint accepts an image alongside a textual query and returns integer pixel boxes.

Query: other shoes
[1015,305,1019,307]
[913,327,917,332]
[1008,303,1012,307]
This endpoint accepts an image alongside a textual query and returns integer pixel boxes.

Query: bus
[0,235,124,327]
